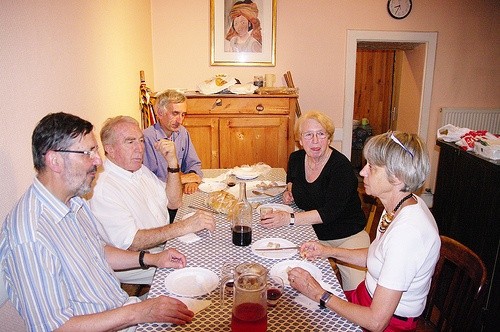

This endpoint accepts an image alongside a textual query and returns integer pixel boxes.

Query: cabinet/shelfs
[183,94,294,169]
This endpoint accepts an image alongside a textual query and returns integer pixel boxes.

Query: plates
[198,182,227,193]
[251,237,297,259]
[256,204,294,216]
[230,180,287,201]
[164,267,219,297]
[271,260,322,288]
[235,168,258,179]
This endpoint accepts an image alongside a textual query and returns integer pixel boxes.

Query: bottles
[232,183,252,246]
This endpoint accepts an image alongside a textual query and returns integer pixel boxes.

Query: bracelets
[139,250,150,270]
[189,170,196,173]
[167,164,180,173]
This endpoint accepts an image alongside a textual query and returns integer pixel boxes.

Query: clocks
[387,0,412,20]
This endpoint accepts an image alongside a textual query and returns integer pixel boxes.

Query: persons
[260,111,371,291]
[287,130,441,332]
[81,115,216,301]
[0,111,194,332]
[142,89,203,224]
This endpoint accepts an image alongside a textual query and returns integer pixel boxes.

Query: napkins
[173,296,212,314]
[202,173,225,183]
[295,281,340,312]
[176,232,198,245]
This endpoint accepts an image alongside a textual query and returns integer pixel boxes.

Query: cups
[226,174,236,187]
[265,74,273,87]
[260,208,274,221]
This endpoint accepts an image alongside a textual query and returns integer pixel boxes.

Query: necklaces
[378,194,413,232]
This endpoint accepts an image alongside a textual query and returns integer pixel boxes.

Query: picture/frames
[209,0,277,67]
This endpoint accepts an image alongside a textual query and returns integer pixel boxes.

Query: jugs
[221,262,271,332]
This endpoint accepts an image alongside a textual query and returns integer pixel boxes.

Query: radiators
[439,107,500,134]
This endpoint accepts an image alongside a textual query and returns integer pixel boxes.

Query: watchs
[290,213,294,225]
[318,291,333,308]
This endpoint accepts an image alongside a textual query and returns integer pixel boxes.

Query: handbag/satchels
[437,123,471,142]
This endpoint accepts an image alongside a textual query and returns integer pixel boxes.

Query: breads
[207,191,237,214]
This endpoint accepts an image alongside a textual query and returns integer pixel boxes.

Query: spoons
[252,191,275,197]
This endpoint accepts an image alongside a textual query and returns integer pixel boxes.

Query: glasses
[42,146,100,159]
[301,130,328,139]
[386,129,414,157]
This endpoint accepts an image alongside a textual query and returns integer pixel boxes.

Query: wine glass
[267,277,284,307]
[222,261,238,297]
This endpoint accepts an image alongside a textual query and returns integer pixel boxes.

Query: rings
[292,278,294,282]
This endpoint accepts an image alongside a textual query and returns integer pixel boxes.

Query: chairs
[426,236,486,332]
[329,190,384,269]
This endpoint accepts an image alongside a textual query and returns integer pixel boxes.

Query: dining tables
[135,168,363,332]
